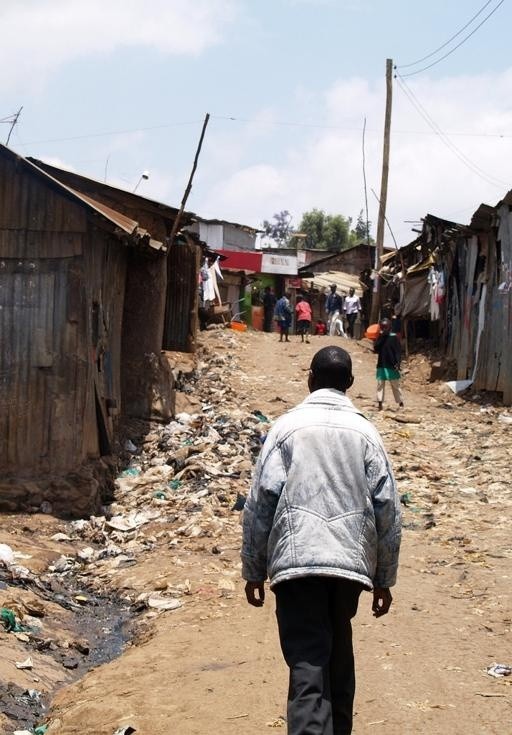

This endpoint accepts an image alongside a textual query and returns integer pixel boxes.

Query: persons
[314,320,326,335]
[272,291,294,342]
[343,287,362,339]
[294,297,312,344]
[374,317,403,410]
[240,346,401,735]
[325,284,343,337]
[263,287,274,332]
